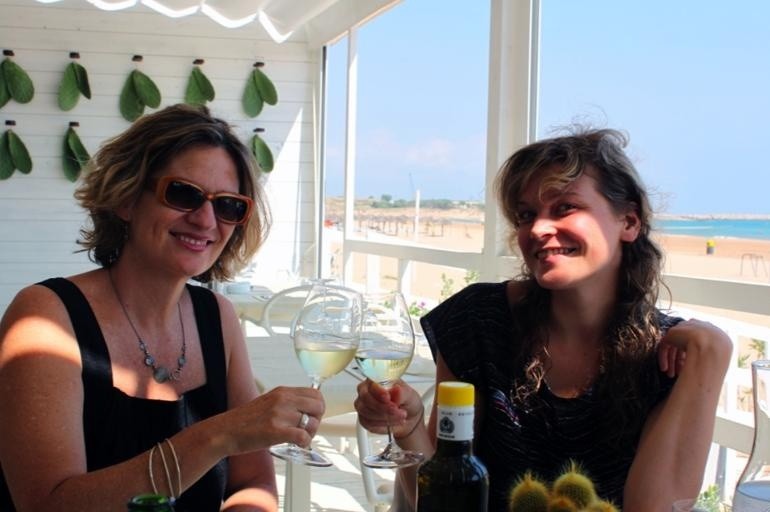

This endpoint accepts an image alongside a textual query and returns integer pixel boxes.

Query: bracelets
[153,440,176,502]
[392,407,425,440]
[148,442,157,504]
[164,436,183,499]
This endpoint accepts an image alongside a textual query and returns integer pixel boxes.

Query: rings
[299,413,310,430]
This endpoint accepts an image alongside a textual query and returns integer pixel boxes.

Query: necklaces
[107,264,188,383]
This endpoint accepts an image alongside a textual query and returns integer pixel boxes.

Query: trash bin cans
[706,240,714,254]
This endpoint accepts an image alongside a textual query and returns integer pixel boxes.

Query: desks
[216,286,436,512]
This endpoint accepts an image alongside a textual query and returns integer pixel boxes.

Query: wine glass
[267,282,361,467]
[353,291,425,469]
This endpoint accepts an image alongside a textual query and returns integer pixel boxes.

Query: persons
[1,99,328,511]
[352,120,736,511]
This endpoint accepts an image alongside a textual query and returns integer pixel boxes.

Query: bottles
[732,359,770,510]
[414,380,489,511]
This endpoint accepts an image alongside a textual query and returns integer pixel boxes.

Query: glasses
[144,176,255,226]
